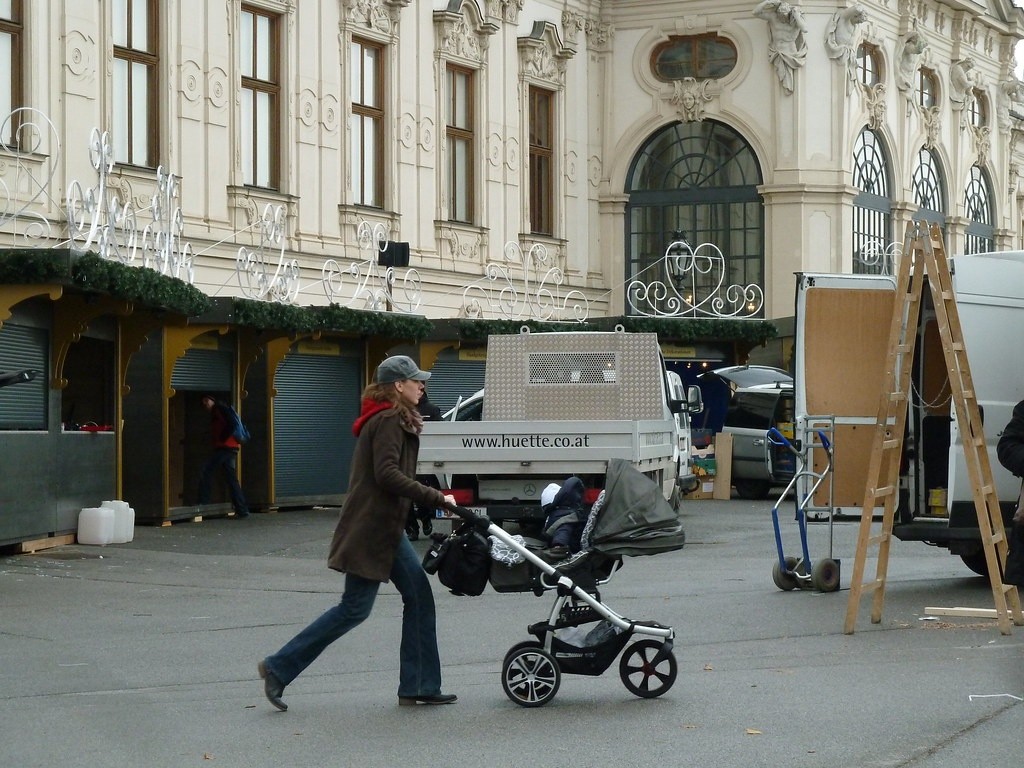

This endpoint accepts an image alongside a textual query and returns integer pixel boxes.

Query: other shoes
[229,513,249,520]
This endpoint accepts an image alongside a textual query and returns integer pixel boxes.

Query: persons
[197,392,250,521]
[404,391,442,541]
[258,355,457,711]
[541,477,585,548]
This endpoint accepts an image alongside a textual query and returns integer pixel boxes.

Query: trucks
[416,323,703,536]
[793,249,1024,584]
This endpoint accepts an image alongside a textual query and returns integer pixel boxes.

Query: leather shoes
[399,692,457,706]
[258,659,288,712]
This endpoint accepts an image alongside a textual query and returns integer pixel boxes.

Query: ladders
[842,220,1024,637]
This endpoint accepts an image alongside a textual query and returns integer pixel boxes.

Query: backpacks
[216,405,250,446]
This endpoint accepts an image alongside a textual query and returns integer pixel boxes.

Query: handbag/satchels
[422,516,493,597]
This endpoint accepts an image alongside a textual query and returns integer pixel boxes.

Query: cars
[683,364,797,500]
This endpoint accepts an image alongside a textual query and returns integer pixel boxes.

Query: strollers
[411,453,687,708]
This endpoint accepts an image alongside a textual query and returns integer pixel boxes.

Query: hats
[377,355,432,384]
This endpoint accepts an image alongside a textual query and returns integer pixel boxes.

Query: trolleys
[764,426,843,596]
